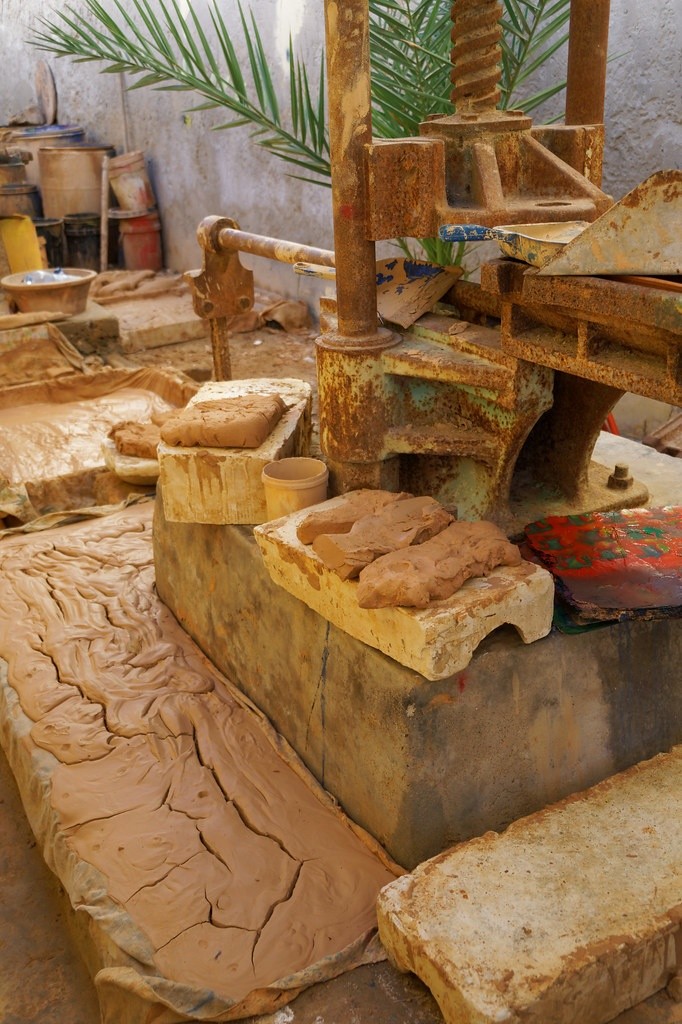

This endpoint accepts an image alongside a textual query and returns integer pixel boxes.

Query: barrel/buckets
[0,124,163,274]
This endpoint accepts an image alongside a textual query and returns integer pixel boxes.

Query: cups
[261,458,329,521]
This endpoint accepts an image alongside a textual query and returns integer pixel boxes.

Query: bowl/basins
[2,266,99,316]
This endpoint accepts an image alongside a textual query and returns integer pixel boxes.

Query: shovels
[292,256,466,329]
[437,218,590,268]
